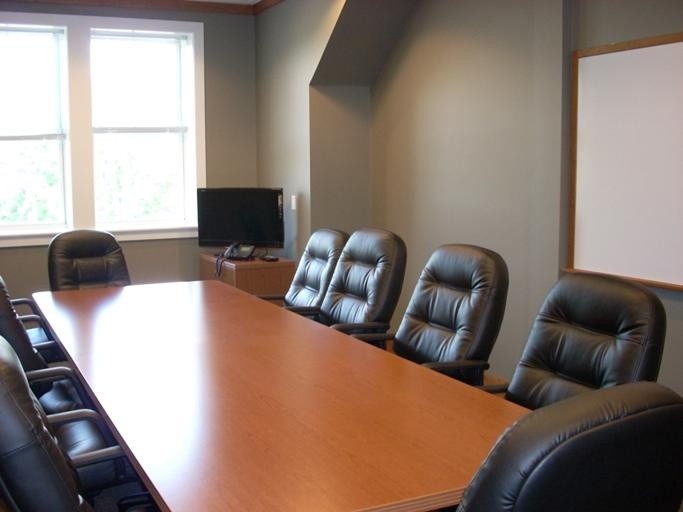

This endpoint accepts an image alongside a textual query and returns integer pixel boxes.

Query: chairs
[456,379,683,511]
[290,227,407,348]
[473,270,667,411]
[48,229,133,292]
[0,273,161,511]
[350,242,509,384]
[253,227,349,312]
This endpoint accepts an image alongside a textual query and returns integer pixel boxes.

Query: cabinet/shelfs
[200,251,296,307]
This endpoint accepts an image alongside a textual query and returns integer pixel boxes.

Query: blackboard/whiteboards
[561,31,683,292]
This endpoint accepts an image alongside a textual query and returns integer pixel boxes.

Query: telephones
[224,240,255,260]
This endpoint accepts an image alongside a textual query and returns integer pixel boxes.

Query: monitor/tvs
[197,187,284,259]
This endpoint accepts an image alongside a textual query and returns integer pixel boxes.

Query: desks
[31,280,533,511]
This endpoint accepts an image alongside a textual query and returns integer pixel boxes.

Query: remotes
[260,256,279,261]
